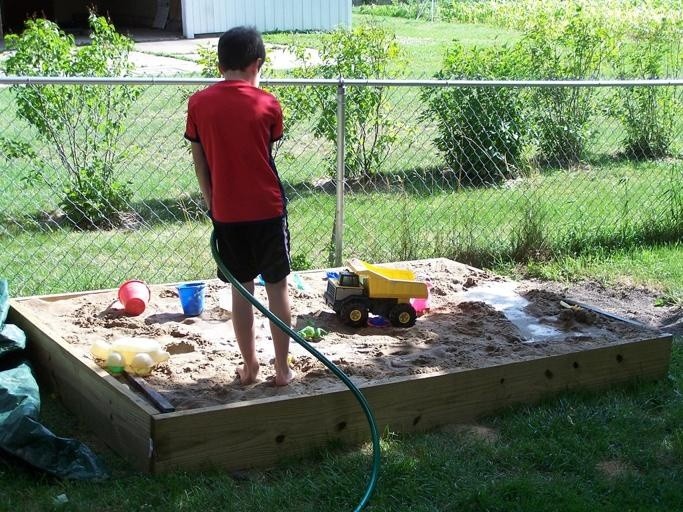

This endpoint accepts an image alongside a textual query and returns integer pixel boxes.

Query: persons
[185,26,298,386]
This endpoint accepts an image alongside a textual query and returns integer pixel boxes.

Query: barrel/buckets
[117,278,152,316]
[174,282,207,317]
